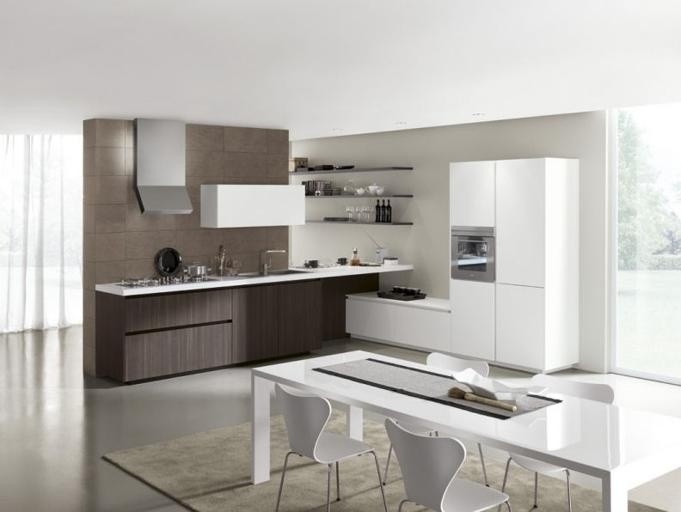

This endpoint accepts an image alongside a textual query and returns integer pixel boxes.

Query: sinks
[236,268,314,278]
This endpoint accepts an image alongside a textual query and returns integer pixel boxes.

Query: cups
[356,185,385,196]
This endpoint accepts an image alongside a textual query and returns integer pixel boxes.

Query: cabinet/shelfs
[96,284,232,384]
[493,157,576,375]
[323,271,381,340]
[447,161,492,228]
[232,279,322,365]
[344,292,449,356]
[200,184,306,229]
[449,279,494,362]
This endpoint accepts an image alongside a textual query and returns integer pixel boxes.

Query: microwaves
[449,224,498,284]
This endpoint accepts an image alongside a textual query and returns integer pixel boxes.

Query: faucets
[259,248,286,273]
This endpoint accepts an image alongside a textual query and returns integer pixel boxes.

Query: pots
[187,264,207,278]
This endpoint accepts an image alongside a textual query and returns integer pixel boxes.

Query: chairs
[385,417,512,511]
[277,386,389,511]
[383,351,489,487]
[499,375,613,512]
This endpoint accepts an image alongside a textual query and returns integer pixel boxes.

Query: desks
[250,345,681,512]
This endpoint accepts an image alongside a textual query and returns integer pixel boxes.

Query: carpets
[99,411,667,512]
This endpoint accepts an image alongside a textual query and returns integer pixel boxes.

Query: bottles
[375,199,392,224]
[342,179,355,196]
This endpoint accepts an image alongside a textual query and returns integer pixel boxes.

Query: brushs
[448,386,517,414]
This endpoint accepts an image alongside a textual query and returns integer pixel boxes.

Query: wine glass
[345,206,374,223]
